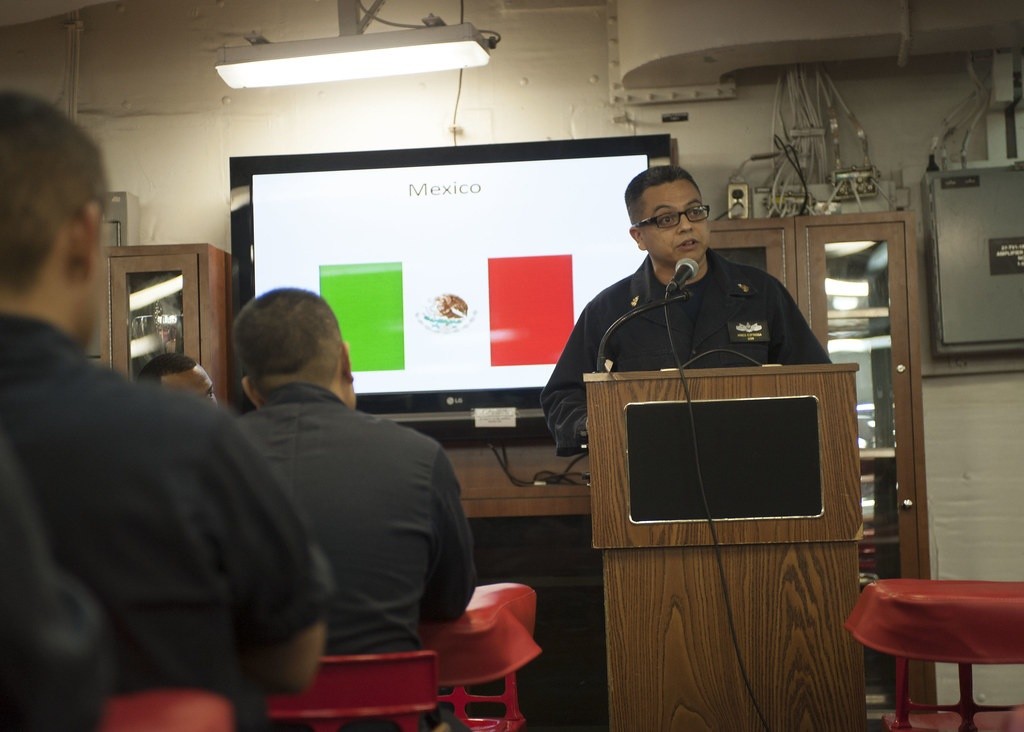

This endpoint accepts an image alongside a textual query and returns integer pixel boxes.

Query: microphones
[666,257,699,294]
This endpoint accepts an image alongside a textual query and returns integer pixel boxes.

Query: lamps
[213,0,491,89]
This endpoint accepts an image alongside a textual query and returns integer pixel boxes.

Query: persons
[135,353,219,410]
[227,286,476,732]
[537,165,835,458]
[0,86,327,732]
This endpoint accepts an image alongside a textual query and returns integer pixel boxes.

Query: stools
[420,584,543,732]
[844,579,1024,732]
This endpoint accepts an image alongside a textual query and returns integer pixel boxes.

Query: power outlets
[727,183,749,219]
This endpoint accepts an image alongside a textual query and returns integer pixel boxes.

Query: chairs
[268,651,438,732]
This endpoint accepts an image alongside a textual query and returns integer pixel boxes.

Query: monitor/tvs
[229,134,674,451]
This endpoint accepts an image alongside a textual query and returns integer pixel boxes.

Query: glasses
[630,204,710,228]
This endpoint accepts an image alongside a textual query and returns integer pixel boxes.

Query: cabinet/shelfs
[89,241,230,412]
[707,210,932,582]
